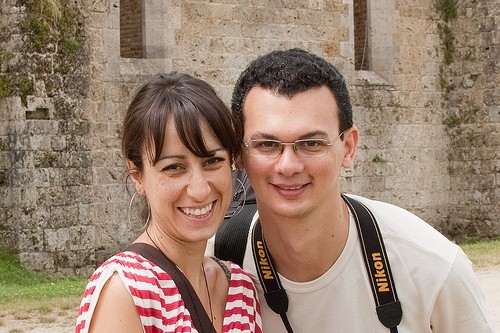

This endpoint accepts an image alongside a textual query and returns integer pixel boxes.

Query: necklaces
[145,228,214,333]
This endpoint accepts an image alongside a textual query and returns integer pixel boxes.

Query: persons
[204,47,493,333]
[76,70,262,333]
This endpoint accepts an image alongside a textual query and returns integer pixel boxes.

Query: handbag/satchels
[225,169,257,218]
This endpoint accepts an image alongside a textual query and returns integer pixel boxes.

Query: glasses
[241,132,344,157]
